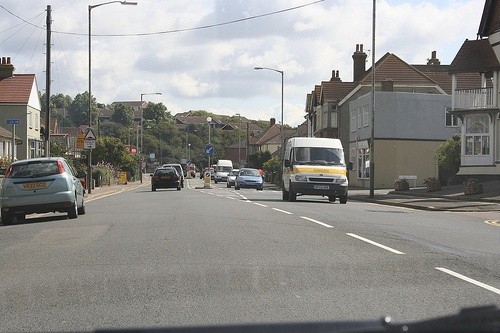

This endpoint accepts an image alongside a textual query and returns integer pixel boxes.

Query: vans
[214,160,234,184]
[282,137,354,204]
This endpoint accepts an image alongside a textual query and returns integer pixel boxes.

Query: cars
[226,169,243,188]
[234,168,264,191]
[163,164,184,189]
[200,165,216,180]
[150,167,182,191]
[0,156,85,224]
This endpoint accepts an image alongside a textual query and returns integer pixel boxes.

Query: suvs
[183,164,196,178]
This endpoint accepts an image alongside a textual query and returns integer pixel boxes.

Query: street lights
[236,112,241,170]
[87,0,138,198]
[140,92,162,155]
[254,66,284,164]
[188,143,191,162]
[207,117,212,172]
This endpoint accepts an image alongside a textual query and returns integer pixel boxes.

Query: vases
[395,184,409,191]
[426,184,441,192]
[464,184,483,195]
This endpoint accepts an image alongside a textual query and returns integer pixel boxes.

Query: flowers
[394,178,408,184]
[463,178,480,188]
[423,176,441,184]
